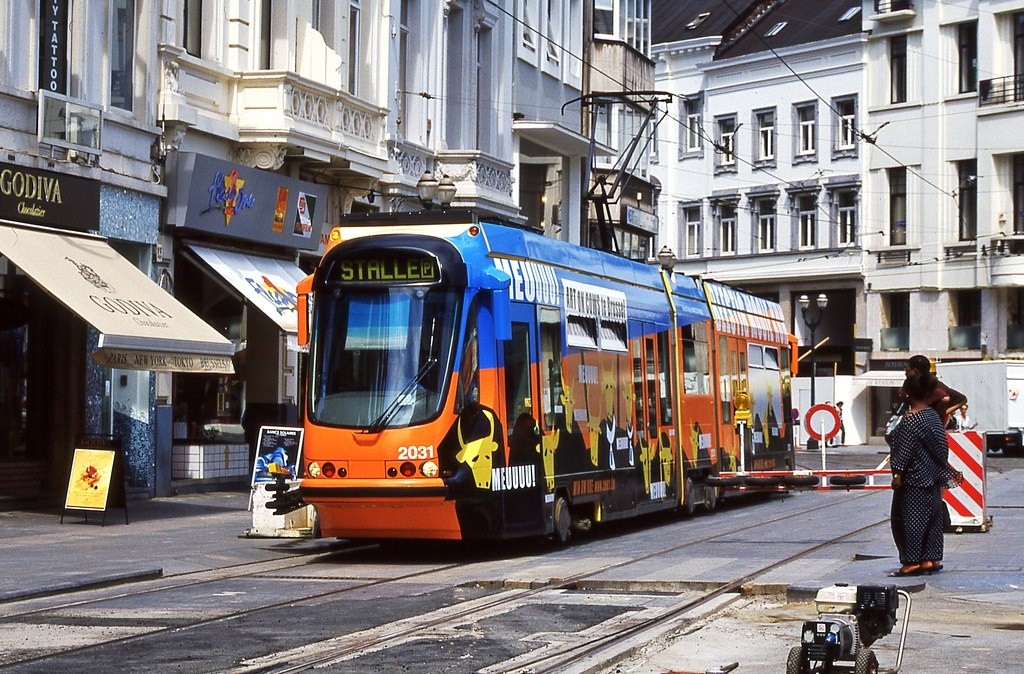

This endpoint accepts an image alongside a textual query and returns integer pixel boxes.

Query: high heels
[931,559,943,571]
[888,559,934,576]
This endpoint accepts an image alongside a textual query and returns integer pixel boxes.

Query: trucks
[936,359,1024,456]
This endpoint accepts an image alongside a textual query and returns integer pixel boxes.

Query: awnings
[182,244,315,351]
[0,226,234,373]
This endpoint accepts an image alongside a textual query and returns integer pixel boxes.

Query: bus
[266,89,801,563]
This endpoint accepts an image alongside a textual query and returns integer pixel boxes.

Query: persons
[886,355,978,577]
[242,382,287,496]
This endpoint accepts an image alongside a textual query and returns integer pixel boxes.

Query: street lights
[799,294,829,452]
